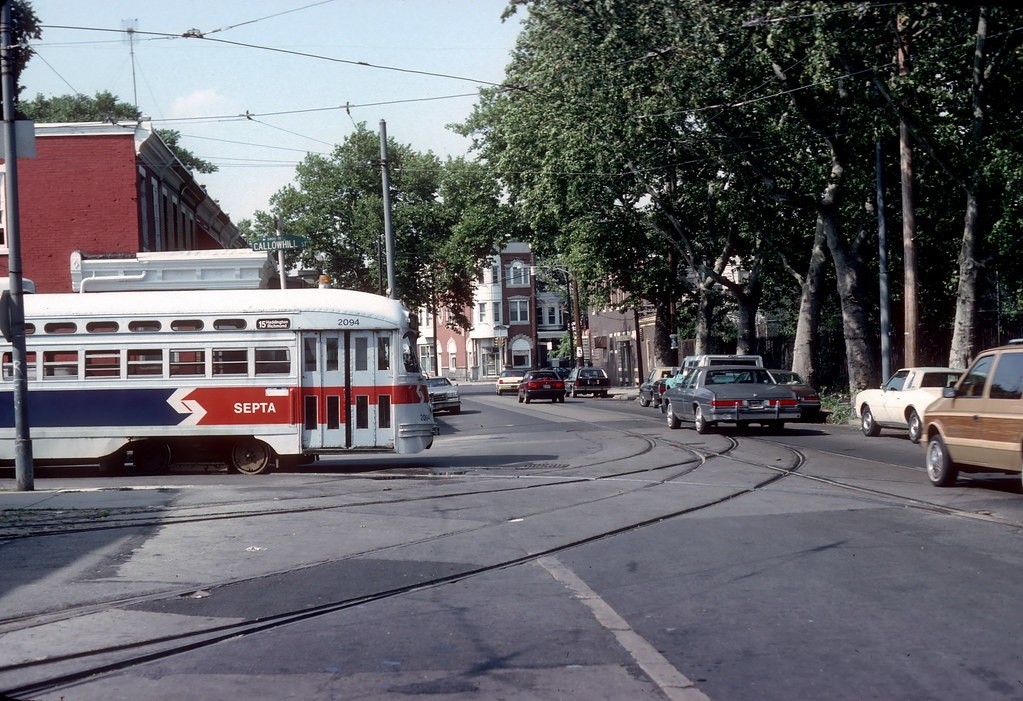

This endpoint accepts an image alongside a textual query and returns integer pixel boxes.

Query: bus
[0,246,441,474]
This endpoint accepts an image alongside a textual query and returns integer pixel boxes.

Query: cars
[425,377,462,415]
[543,366,570,380]
[564,366,611,398]
[658,364,802,434]
[638,365,682,409]
[768,368,822,418]
[517,370,566,404]
[495,369,527,396]
[854,368,968,443]
[922,338,1022,487]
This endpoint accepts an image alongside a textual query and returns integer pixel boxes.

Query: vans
[666,353,764,391]
